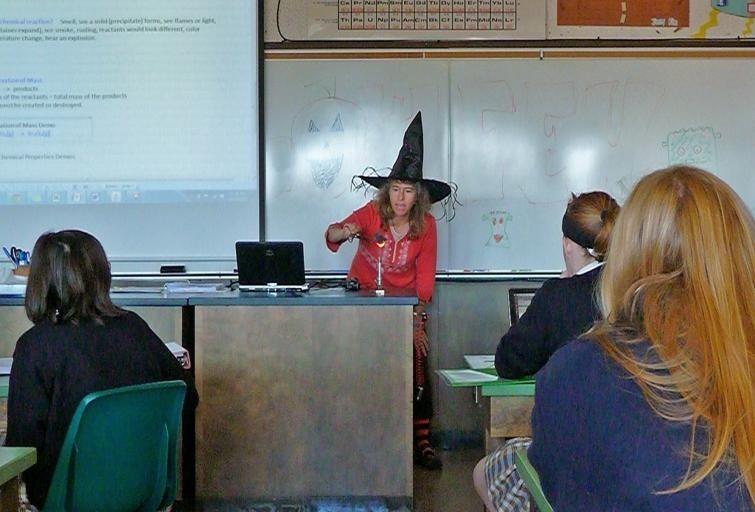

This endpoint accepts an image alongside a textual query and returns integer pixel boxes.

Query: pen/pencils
[377,256,382,289]
[3,247,19,269]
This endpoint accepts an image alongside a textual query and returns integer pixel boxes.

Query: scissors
[11,247,30,263]
[344,226,369,243]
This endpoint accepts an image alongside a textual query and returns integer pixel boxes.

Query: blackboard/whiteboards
[108,37,755,283]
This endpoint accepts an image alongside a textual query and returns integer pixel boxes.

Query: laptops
[507,287,540,327]
[235,241,316,291]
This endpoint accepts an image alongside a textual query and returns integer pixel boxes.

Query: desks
[0,447,37,512]
[464,352,535,455]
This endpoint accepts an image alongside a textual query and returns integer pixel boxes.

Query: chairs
[43,379,186,512]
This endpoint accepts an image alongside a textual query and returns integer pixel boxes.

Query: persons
[472,190,621,511]
[529,165,755,511]
[4,229,198,512]
[326,179,443,471]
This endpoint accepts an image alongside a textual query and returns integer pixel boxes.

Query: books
[165,281,226,294]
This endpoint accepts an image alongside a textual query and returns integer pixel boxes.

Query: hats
[358,110,451,204]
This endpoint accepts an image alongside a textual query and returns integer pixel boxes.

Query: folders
[164,342,191,369]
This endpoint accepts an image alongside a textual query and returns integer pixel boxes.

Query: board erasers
[160,266,186,273]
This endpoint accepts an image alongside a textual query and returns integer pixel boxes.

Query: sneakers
[413,444,442,469]
